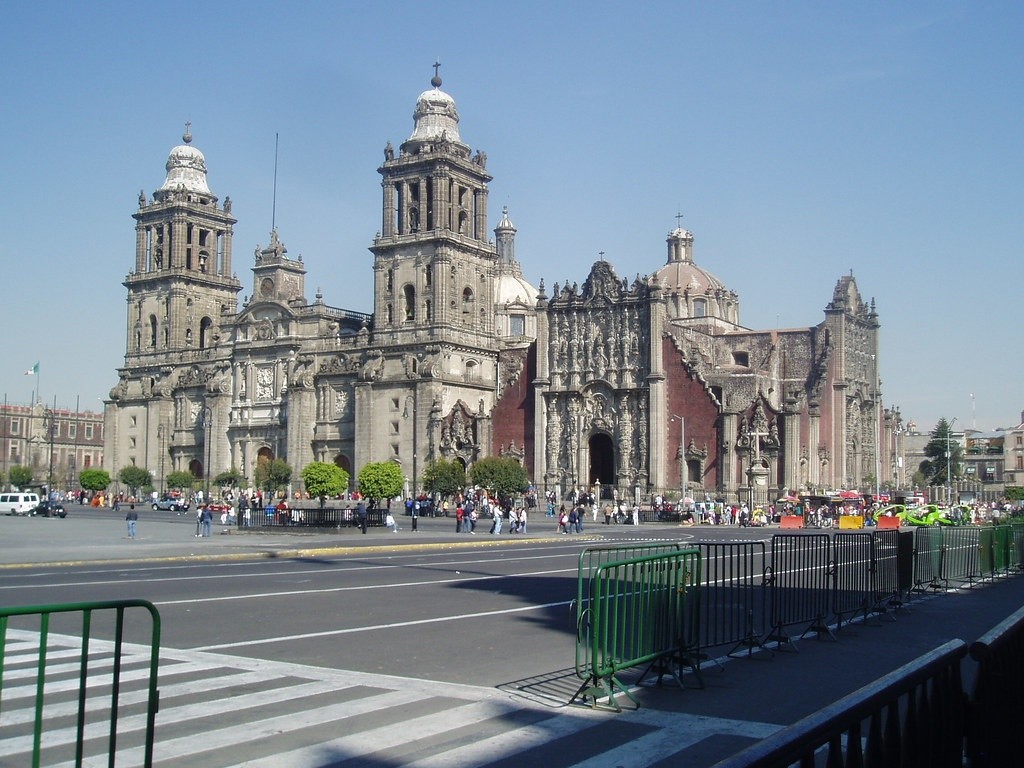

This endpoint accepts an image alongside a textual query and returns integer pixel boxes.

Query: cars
[28,500,67,518]
[151,497,180,511]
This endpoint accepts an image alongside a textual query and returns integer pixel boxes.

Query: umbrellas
[777,496,799,503]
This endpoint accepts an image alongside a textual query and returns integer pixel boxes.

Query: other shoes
[562,533,566,534]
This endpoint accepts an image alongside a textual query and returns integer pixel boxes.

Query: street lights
[563,417,576,509]
[201,406,213,504]
[671,414,685,507]
[401,380,417,502]
[947,417,957,507]
[43,408,55,502]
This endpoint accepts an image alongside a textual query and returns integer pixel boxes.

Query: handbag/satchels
[562,516,569,523]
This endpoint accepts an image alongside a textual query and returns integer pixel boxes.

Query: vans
[0,493,40,516]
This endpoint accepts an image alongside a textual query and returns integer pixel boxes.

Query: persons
[650,493,1016,527]
[52,483,628,539]
